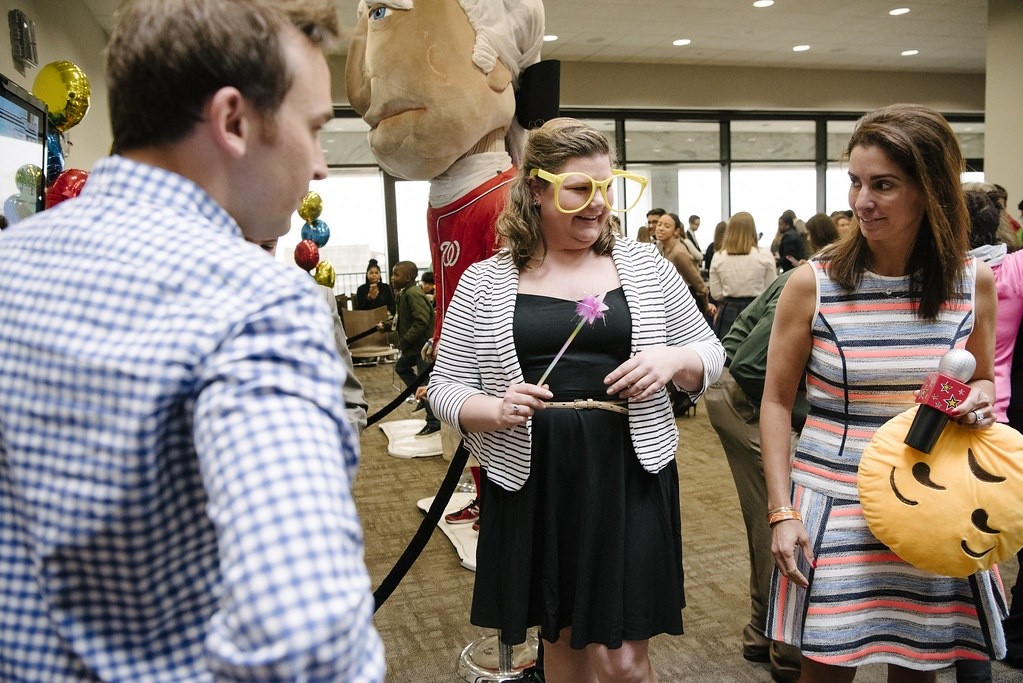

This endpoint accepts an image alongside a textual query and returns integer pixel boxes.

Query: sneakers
[416,424,441,437]
[445,497,482,524]
[472,518,481,531]
[413,402,425,413]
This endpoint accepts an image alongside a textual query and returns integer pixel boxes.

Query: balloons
[32,61,90,130]
[298,192,322,225]
[293,239,319,270]
[302,220,330,246]
[312,261,335,288]
[2,132,89,222]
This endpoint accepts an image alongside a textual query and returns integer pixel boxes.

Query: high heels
[671,395,696,418]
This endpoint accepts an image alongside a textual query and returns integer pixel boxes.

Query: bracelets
[767,506,802,525]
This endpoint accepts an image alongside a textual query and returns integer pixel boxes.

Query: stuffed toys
[856,403,1022,576]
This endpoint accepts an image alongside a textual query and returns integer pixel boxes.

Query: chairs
[341,305,403,395]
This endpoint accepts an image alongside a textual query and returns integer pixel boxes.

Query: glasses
[529,169,648,213]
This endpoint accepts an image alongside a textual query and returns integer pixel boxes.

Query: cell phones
[370,284,377,291]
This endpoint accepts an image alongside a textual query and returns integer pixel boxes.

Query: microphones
[904,349,977,455]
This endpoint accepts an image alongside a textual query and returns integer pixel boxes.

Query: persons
[429,116,727,683]
[421,273,435,295]
[704,182,1022,682]
[376,260,433,414]
[2,0,387,683]
[357,259,396,316]
[610,207,717,417]
[757,104,1013,683]
[344,0,544,534]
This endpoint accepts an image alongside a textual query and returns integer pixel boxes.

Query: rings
[974,410,983,423]
[513,404,519,412]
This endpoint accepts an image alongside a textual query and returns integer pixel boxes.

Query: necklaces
[873,268,910,300]
